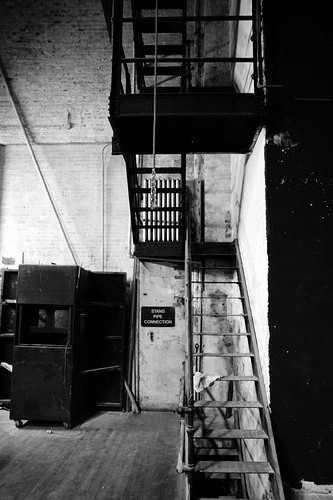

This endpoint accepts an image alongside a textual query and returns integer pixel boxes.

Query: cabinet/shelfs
[9,263,93,430]
